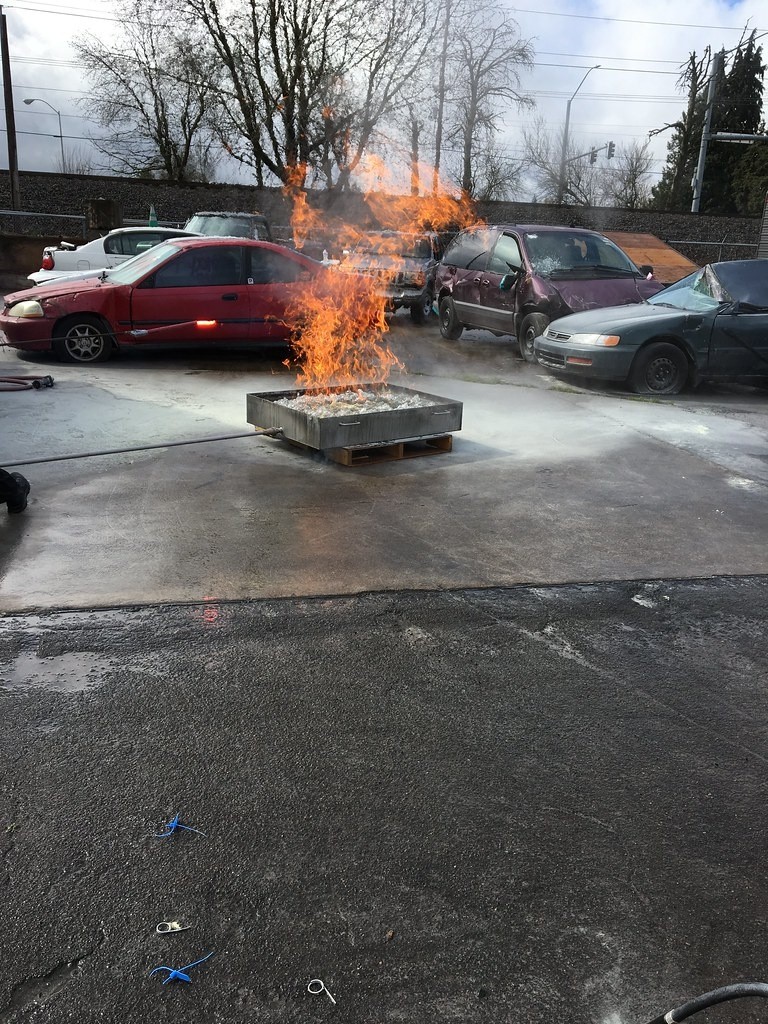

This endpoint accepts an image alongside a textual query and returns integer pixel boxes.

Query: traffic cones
[148,205,158,226]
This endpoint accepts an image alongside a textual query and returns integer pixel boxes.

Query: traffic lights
[607,139,616,159]
[590,146,598,164]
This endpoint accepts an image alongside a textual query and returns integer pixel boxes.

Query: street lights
[24,98,66,173]
[557,63,602,203]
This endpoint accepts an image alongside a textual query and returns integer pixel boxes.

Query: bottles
[323,249,328,262]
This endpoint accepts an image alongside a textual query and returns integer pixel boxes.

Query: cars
[185,213,277,245]
[336,229,445,327]
[434,220,667,365]
[0,233,379,364]
[29,226,206,286]
[533,258,768,397]
